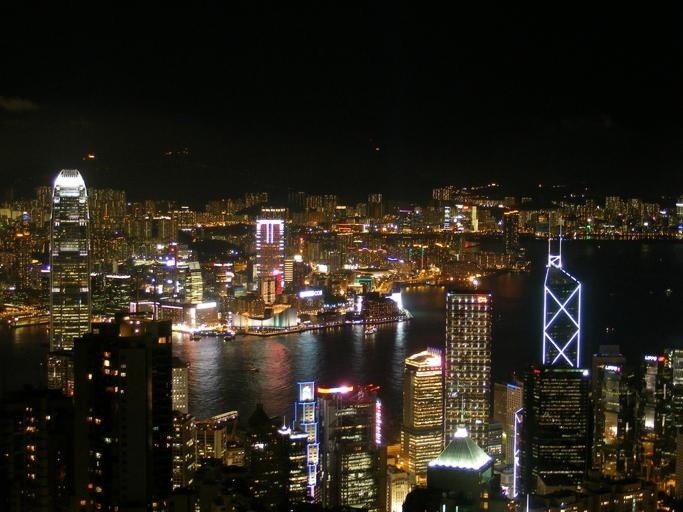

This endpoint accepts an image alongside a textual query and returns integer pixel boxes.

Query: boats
[364,325,378,334]
